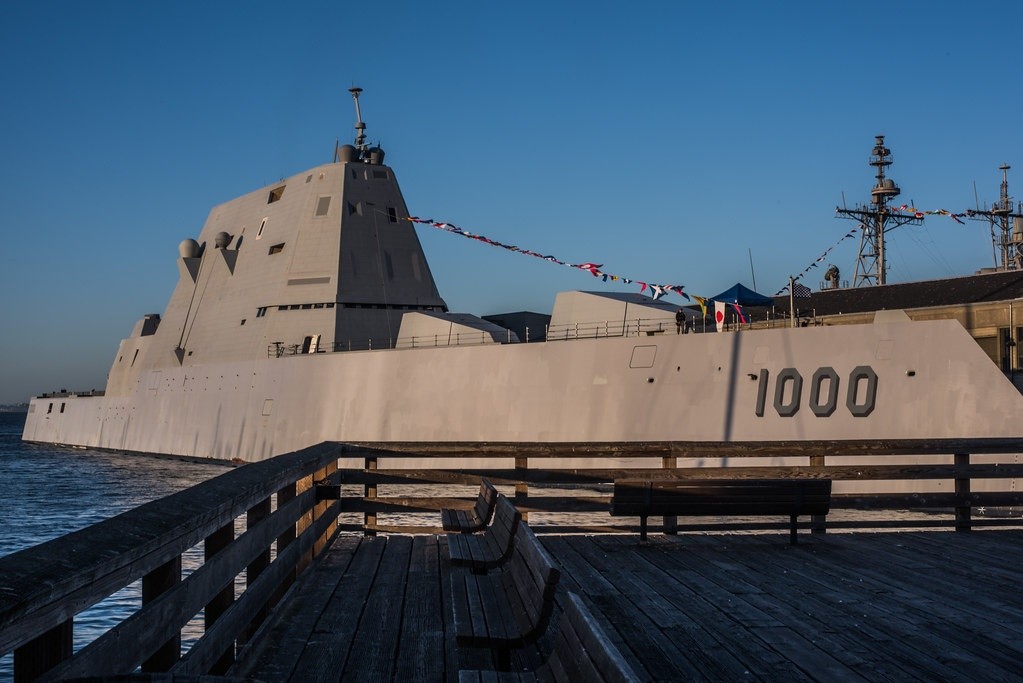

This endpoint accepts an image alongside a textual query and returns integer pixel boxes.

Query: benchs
[457,589,643,683]
[448,520,560,676]
[441,477,497,534]
[446,494,523,575]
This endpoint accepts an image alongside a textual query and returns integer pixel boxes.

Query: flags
[635,281,708,317]
[714,300,725,333]
[403,216,634,284]
[727,302,750,323]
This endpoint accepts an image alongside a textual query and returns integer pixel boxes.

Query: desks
[604,473,835,548]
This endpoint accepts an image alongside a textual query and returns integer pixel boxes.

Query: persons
[676,308,686,335]
